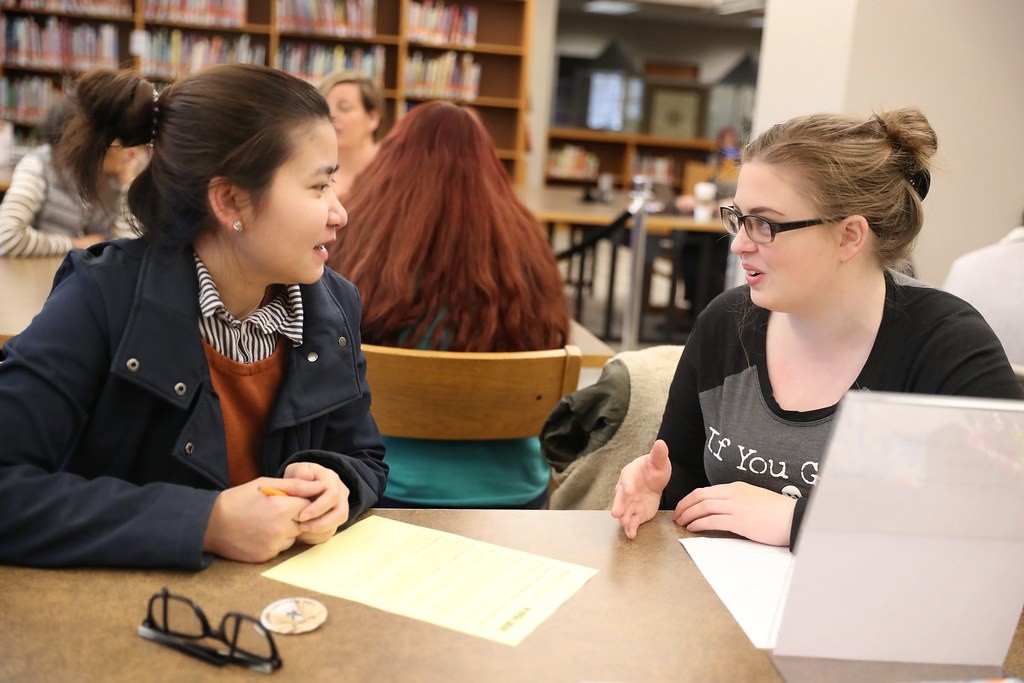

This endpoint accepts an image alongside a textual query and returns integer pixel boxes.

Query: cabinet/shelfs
[0,0,537,196]
[544,128,745,233]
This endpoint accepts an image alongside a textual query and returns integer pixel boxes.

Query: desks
[0,252,615,368]
[540,191,734,346]
[1,510,1024,683]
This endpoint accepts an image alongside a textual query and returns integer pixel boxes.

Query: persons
[0,63,390,575]
[0,136,153,259]
[656,172,736,334]
[315,69,385,200]
[612,105,1024,554]
[716,126,742,163]
[943,207,1024,374]
[324,100,571,509]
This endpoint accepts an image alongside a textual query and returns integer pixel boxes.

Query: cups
[693,182,717,223]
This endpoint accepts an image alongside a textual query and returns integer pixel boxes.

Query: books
[0,0,487,192]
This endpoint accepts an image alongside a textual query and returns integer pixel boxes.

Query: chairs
[359,343,582,510]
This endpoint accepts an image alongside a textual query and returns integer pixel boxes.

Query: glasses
[137,586,282,675]
[720,206,881,244]
